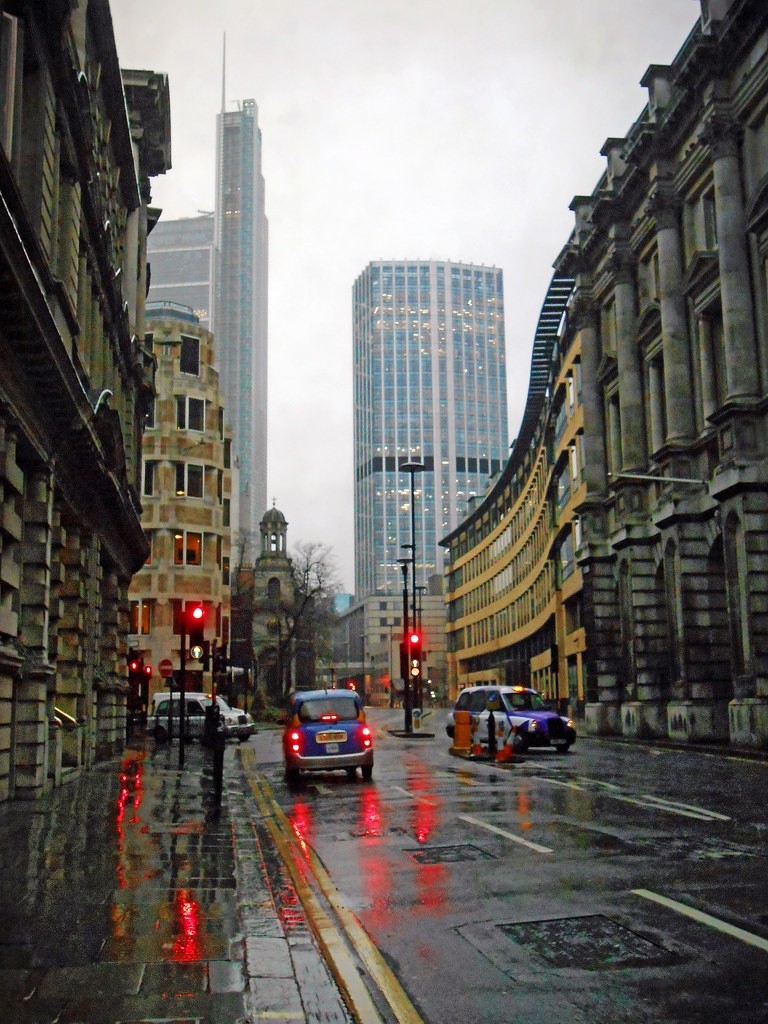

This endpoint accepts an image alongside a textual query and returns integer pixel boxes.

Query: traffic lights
[189,606,204,661]
[410,632,419,677]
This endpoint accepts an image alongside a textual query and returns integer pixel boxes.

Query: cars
[277,688,375,781]
[445,685,577,753]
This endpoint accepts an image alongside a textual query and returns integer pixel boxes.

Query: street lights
[398,461,428,712]
[414,585,427,716]
[384,558,436,739]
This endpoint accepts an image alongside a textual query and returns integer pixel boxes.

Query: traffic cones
[469,724,488,761]
[494,724,517,764]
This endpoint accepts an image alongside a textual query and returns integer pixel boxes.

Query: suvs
[145,691,256,744]
